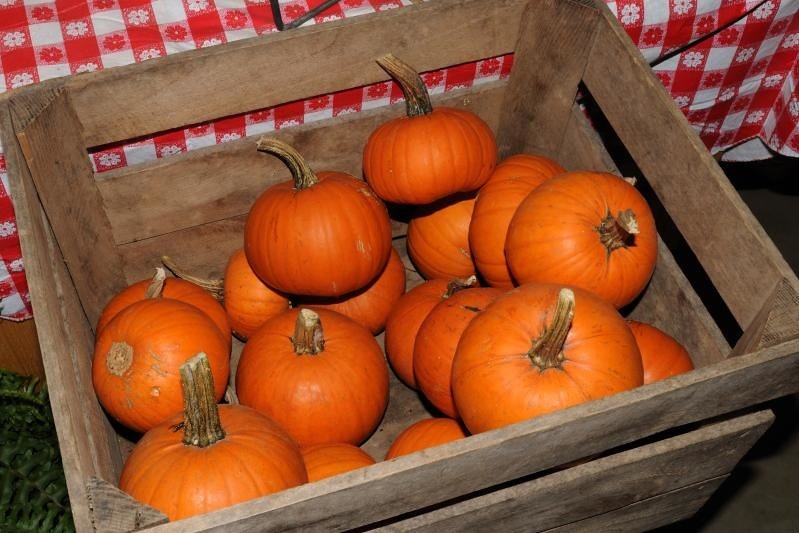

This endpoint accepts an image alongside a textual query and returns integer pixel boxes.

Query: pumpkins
[92,53,694,520]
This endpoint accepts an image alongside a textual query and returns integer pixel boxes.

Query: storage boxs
[0,0,799,533]
